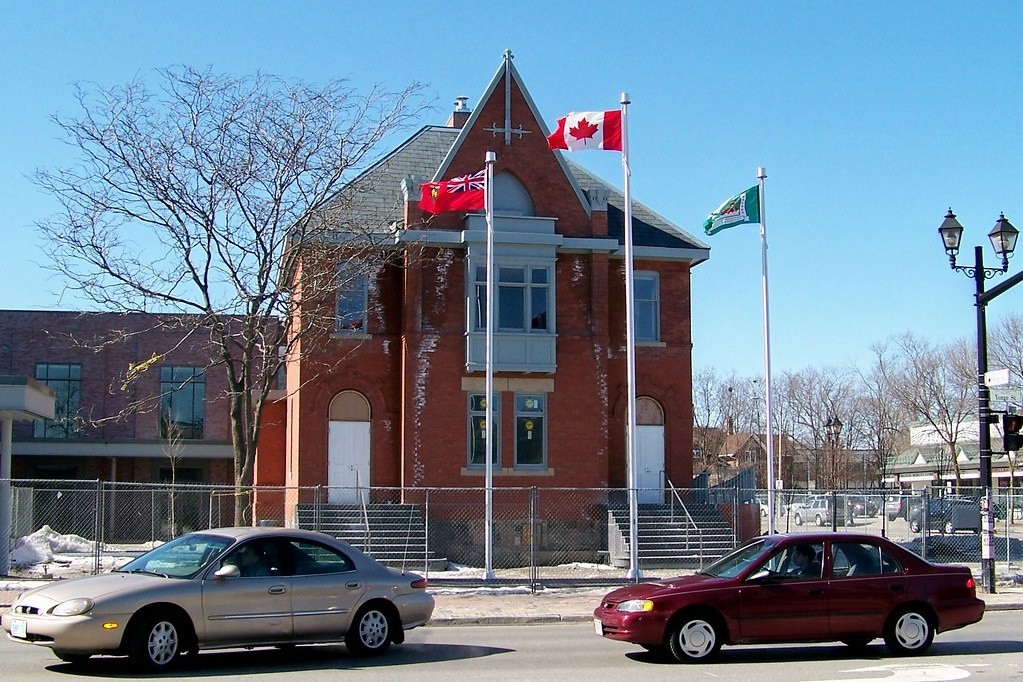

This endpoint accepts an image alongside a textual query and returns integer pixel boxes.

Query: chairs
[817,550,834,570]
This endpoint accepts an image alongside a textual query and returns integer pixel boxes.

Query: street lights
[938,207,1023,594]
[824,416,842,532]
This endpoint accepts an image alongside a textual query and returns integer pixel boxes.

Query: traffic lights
[1003,411,1023,453]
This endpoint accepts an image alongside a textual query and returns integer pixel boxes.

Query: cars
[756,499,785,517]
[594,534,986,663]
[911,500,982,534]
[847,497,877,517]
[794,499,845,526]
[1,526,434,672]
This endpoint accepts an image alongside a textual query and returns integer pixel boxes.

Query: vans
[803,495,843,506]
[886,495,922,520]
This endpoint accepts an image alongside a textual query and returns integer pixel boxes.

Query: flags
[704,185,760,235]
[547,109,622,151]
[417,169,485,213]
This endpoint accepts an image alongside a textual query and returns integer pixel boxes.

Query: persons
[222,544,266,576]
[759,545,821,580]
